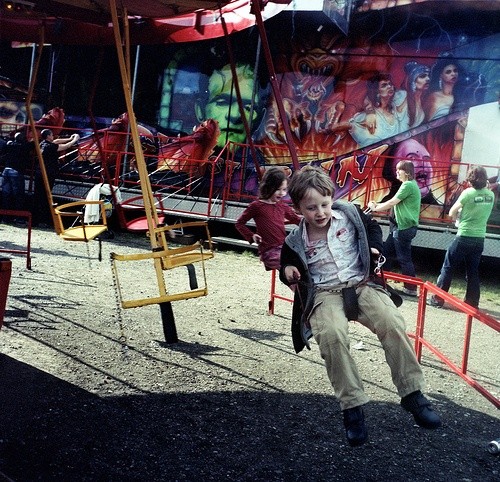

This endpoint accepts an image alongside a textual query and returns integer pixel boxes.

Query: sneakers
[426,299,443,308]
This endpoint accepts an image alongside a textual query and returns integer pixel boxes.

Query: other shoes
[394,288,417,301]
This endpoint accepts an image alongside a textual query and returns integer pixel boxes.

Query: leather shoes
[343,407,368,447]
[401,390,440,429]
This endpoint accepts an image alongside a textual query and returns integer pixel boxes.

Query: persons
[368,160,420,296]
[430,167,495,308]
[2,133,38,227]
[236,168,301,269]
[35,128,79,224]
[280,166,442,448]
